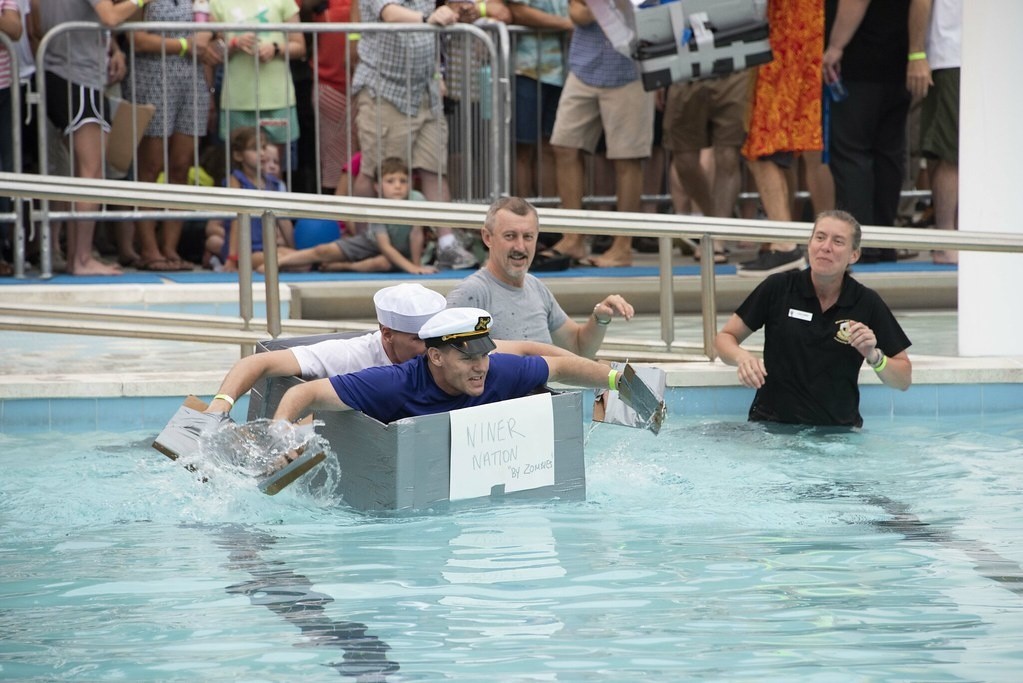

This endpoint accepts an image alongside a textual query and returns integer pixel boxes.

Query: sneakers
[434,238,480,270]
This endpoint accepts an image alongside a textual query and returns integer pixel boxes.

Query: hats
[418,307,497,356]
[373,282,447,334]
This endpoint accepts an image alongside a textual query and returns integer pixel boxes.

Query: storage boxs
[246,335,589,520]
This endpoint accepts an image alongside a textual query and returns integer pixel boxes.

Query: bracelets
[214,394,234,408]
[908,52,926,59]
[349,33,360,40]
[481,2,486,17]
[273,42,280,57]
[867,348,886,372]
[232,37,236,47]
[609,370,618,390]
[593,303,612,326]
[178,37,188,58]
[133,0,144,8]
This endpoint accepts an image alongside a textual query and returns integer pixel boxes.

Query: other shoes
[739,244,808,278]
[734,249,771,268]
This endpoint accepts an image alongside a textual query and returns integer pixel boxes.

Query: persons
[201,285,608,416]
[444,196,634,362]
[0,1,964,275]
[714,210,912,427]
[265,307,622,476]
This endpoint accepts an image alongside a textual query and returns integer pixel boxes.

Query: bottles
[193,0,209,22]
[916,158,932,205]
[824,67,849,101]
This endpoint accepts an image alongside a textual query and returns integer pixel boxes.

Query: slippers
[713,246,729,265]
[539,245,580,265]
[133,256,170,271]
[169,254,193,271]
